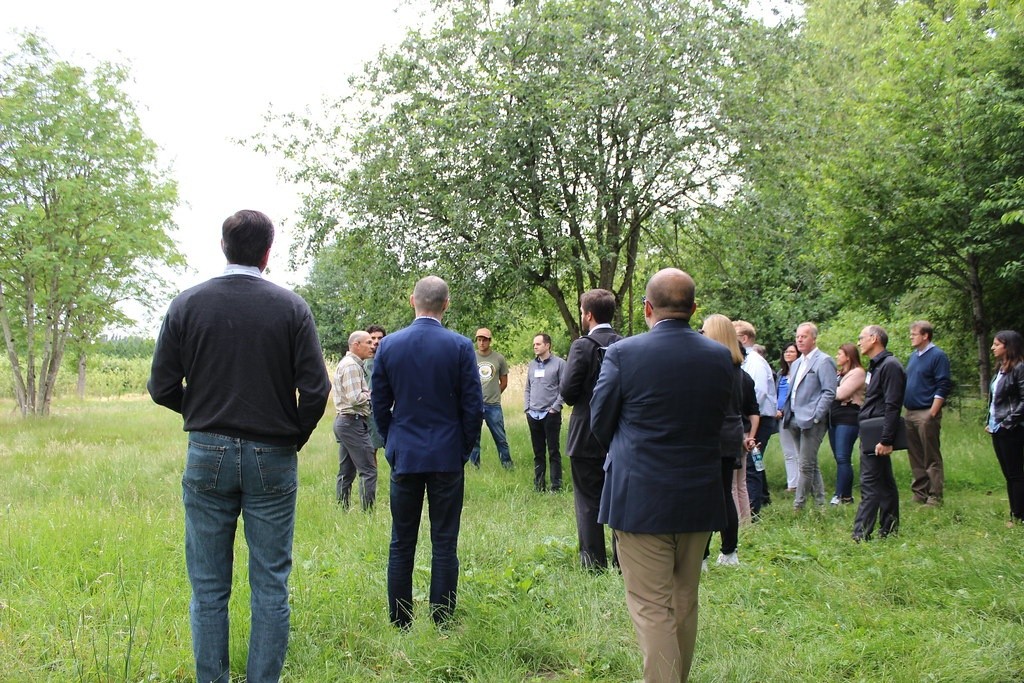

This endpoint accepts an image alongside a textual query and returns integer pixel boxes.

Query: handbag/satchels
[859,417,909,454]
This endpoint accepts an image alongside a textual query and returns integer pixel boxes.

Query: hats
[475,328,492,338]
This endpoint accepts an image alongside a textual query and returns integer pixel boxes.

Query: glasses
[858,335,877,341]
[698,329,705,334]
[642,296,653,310]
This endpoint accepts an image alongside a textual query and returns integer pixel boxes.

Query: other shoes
[840,497,853,505]
[785,488,796,492]
[702,559,708,571]
[714,552,738,566]
[830,496,839,504]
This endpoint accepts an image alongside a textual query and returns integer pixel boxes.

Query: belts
[339,413,365,419]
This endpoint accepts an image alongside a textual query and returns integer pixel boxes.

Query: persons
[560,288,623,573]
[363,325,386,456]
[904,321,950,505]
[985,330,1024,526]
[776,342,802,493]
[783,322,837,509]
[854,325,906,542]
[470,327,512,469]
[370,276,483,633]
[591,267,733,682]
[147,209,332,683]
[332,331,377,516]
[829,343,867,506]
[731,320,778,524]
[524,334,566,493]
[702,309,742,572]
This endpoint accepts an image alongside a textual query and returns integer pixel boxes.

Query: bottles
[750,446,765,472]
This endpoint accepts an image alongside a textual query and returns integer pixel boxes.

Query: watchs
[814,419,819,424]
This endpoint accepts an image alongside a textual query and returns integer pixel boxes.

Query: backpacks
[579,334,619,393]
